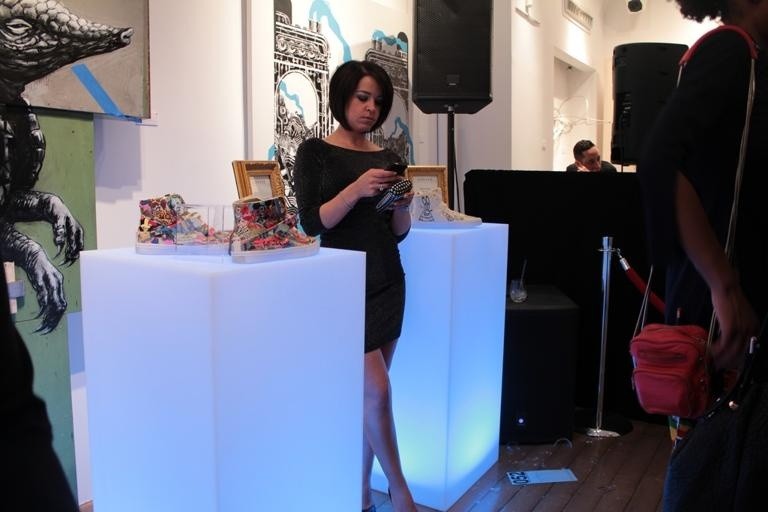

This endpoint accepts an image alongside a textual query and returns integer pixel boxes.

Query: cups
[510,281,528,303]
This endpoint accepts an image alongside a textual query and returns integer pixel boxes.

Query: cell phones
[384,162,408,175]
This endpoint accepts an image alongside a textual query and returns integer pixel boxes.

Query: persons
[644,0,765,510]
[295,60,417,512]
[566,140,615,172]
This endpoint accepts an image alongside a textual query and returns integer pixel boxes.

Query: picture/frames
[231,159,287,200]
[406,165,448,206]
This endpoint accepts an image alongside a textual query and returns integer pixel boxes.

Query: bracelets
[335,188,355,212]
[396,205,411,212]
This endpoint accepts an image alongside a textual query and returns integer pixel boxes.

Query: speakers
[411,0,493,115]
[611,42,689,167]
[499,283,581,446]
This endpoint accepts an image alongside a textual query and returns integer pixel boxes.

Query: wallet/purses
[376,179,412,215]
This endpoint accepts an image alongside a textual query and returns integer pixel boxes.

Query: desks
[506,282,581,316]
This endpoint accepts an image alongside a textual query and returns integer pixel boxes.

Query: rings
[378,182,385,192]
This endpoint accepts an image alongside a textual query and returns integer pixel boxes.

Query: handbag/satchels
[629,323,710,420]
